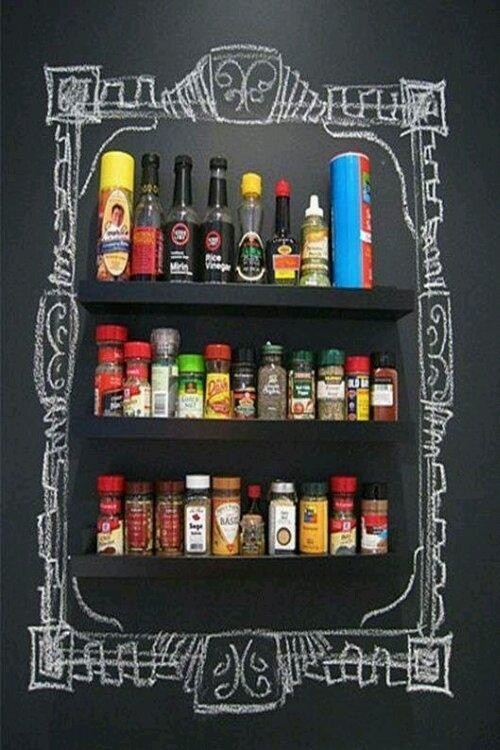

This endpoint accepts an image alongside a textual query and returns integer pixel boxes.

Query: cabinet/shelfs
[67,282,414,579]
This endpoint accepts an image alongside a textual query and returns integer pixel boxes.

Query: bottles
[96,152,134,282]
[162,155,203,283]
[212,476,241,557]
[154,481,184,555]
[203,345,232,418]
[317,350,345,419]
[268,483,297,556]
[184,474,213,558]
[176,354,205,419]
[233,173,268,284]
[330,154,374,289]
[298,483,329,556]
[124,481,154,555]
[93,327,126,418]
[288,351,315,419]
[122,342,151,422]
[95,476,125,555]
[199,156,235,284]
[256,346,288,420]
[345,355,371,420]
[230,348,255,420]
[239,486,267,557]
[328,476,358,555]
[131,154,163,281]
[266,180,302,285]
[361,483,388,555]
[371,353,398,423]
[149,330,179,417]
[301,194,331,287]
